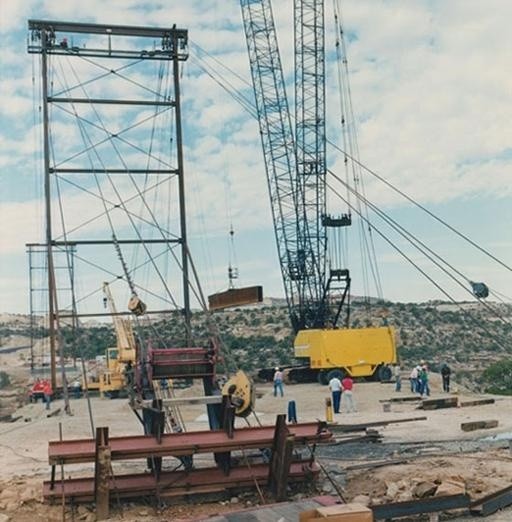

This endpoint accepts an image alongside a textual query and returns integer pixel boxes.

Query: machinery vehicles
[227,1,398,386]
[82,280,137,400]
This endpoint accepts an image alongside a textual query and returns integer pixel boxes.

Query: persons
[273,366,284,397]
[394,362,403,391]
[340,373,357,413]
[440,362,451,393]
[327,372,345,413]
[407,364,430,397]
[26,381,34,403]
[73,377,81,398]
[30,379,46,403]
[42,381,54,411]
